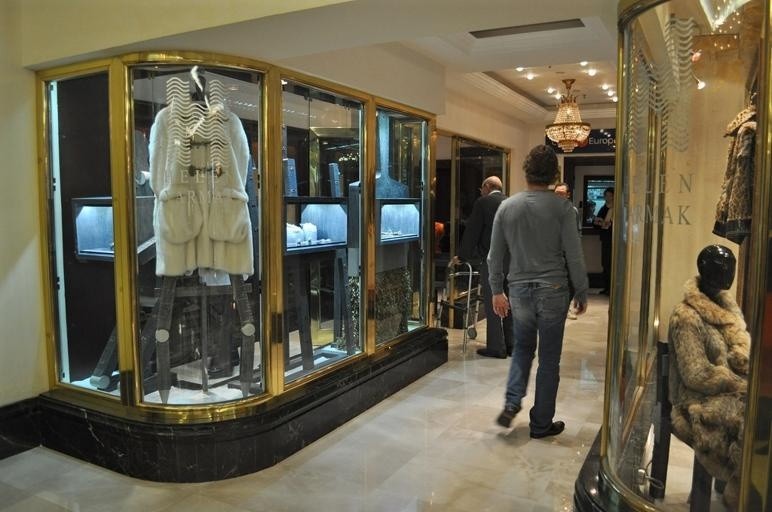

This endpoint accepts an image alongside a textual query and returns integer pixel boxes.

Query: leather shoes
[477,348,507,359]
[531,420,565,438]
[498,406,518,429]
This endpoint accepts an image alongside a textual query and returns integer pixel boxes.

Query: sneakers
[566,309,577,319]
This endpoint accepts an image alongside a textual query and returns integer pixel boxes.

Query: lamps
[544,77,593,154]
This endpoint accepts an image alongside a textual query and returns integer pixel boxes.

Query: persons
[451,176,513,360]
[591,186,614,298]
[484,143,592,443]
[552,182,581,320]
[664,244,751,510]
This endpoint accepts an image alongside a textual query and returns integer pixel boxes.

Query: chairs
[650,337,726,512]
